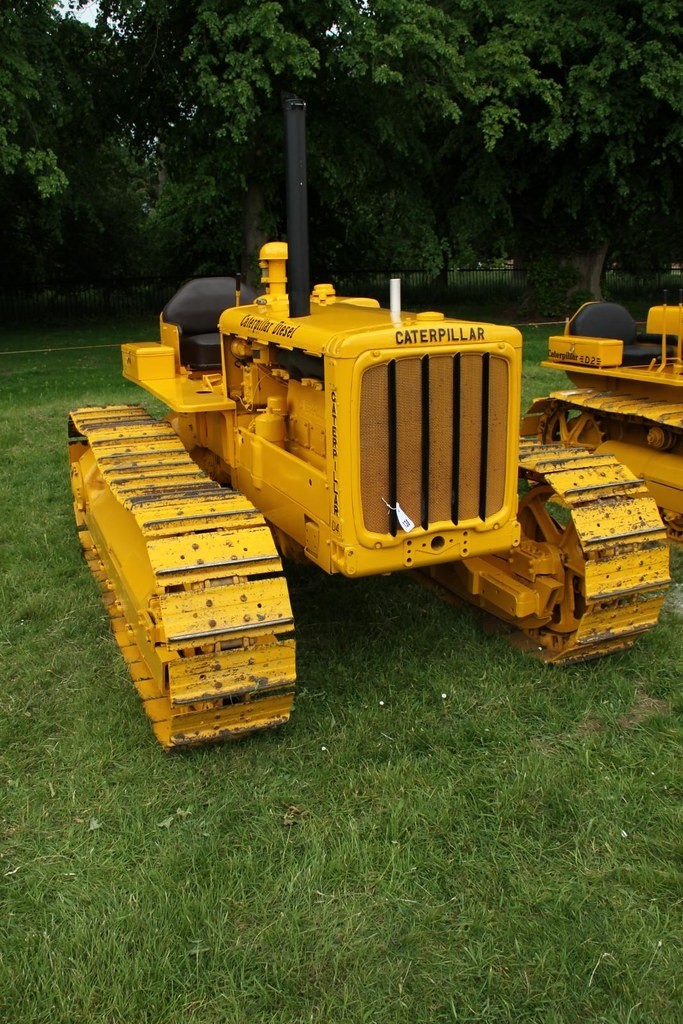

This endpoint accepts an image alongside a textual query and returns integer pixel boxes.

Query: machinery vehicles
[517,302,682,553]
[67,91,669,752]
